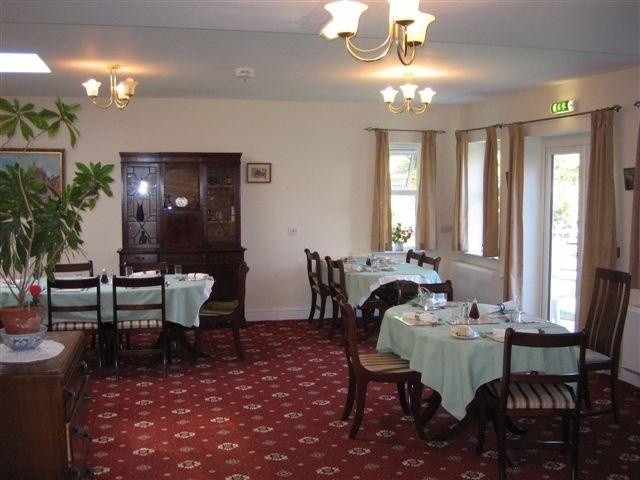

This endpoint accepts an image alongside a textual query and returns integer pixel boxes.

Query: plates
[452,332,479,339]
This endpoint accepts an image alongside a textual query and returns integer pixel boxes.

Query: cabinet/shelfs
[1,322,93,477]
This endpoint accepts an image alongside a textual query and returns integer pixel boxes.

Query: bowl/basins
[1,324,48,354]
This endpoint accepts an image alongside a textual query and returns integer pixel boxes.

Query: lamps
[323,0,438,67]
[378,73,434,115]
[80,60,139,110]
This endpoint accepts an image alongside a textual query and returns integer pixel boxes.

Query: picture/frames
[247,160,274,186]
[0,146,67,201]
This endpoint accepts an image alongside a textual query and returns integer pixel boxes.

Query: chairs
[301,238,443,343]
[1,247,252,381]
[390,281,459,307]
[474,322,592,470]
[319,300,427,442]
[569,266,633,423]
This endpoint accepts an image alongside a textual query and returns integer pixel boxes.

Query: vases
[394,242,404,251]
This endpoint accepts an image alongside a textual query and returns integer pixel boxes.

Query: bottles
[469,299,480,320]
[365,254,378,271]
[164,194,174,209]
[101,269,108,283]
[136,200,145,222]
[138,231,149,245]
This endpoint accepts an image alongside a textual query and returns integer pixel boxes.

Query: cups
[439,298,447,308]
[174,265,183,280]
[451,302,468,325]
[458,325,470,336]
[125,266,133,278]
[425,298,434,312]
[510,311,521,330]
[187,272,204,281]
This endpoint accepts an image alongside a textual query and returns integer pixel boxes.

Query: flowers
[390,222,414,242]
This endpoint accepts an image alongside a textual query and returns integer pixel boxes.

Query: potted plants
[0,93,118,330]
